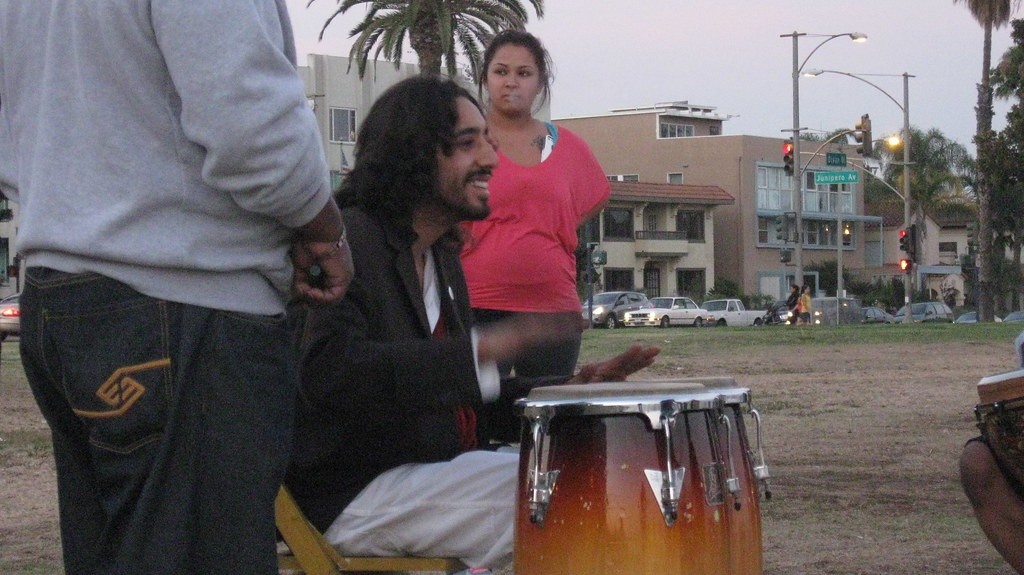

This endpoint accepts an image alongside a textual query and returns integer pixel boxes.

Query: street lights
[801,69,912,324]
[792,31,870,291]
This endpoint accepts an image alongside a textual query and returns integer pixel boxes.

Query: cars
[0,291,22,344]
[764,296,862,325]
[623,296,708,329]
[954,310,1002,324]
[1003,310,1024,323]
[858,306,895,326]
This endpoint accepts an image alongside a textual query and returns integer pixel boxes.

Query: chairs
[275,483,470,575]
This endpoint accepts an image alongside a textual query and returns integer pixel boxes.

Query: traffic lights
[855,112,873,157]
[899,258,912,272]
[784,137,794,177]
[899,226,912,253]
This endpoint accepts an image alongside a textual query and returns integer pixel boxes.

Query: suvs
[893,301,956,328]
[582,291,654,329]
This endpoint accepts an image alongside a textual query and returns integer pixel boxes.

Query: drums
[974,367,1024,494]
[513,382,735,575]
[711,387,772,575]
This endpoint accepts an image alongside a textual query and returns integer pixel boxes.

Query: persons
[786,284,811,324]
[280,73,661,575]
[453,29,610,575]
[963,293,968,308]
[0,0,356,575]
[960,328,1024,575]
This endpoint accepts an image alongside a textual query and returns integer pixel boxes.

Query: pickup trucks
[699,298,769,328]
